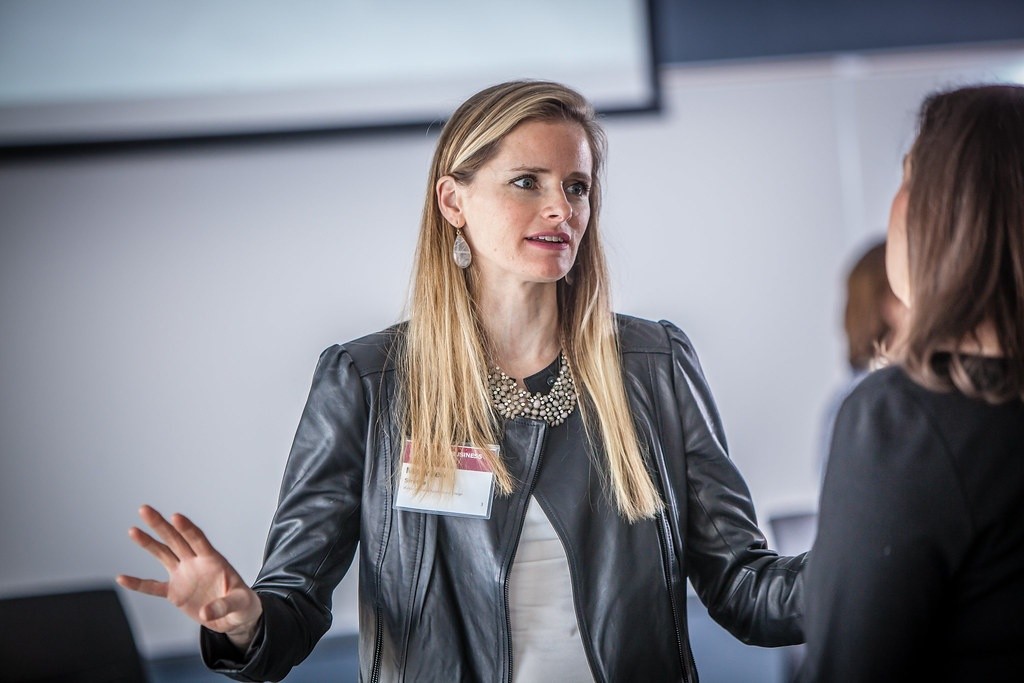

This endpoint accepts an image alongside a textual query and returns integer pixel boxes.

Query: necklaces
[467,318,577,425]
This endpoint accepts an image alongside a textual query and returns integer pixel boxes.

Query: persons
[787,83,1024,682]
[118,80,812,682]
[821,241,910,515]
